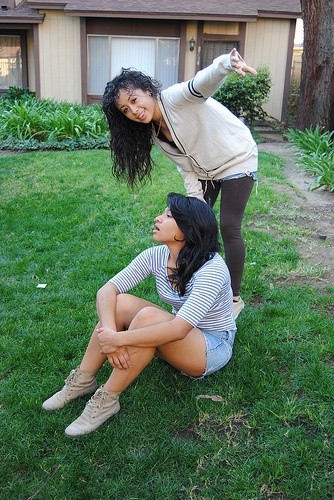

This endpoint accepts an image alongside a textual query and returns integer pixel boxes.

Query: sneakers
[42,365,99,411]
[65,384,122,437]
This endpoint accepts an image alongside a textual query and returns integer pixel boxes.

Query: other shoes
[232,296,245,320]
